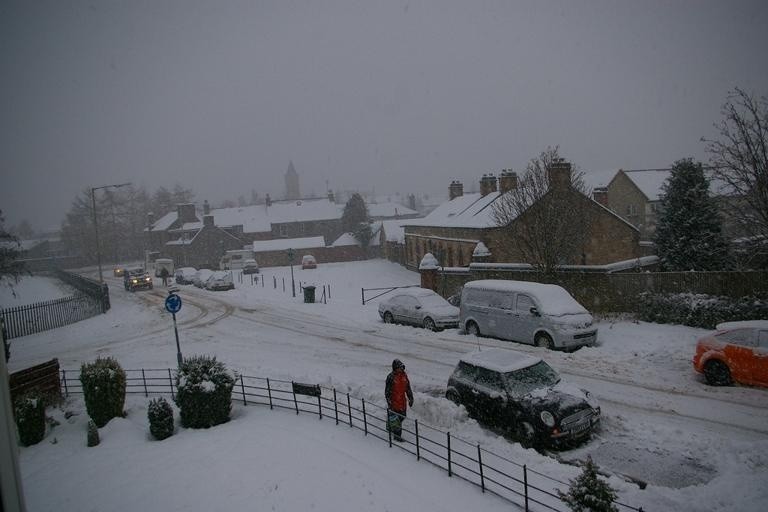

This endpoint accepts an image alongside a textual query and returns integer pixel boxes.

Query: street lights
[91,182,132,280]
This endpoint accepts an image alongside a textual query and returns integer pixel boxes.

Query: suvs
[124,267,153,292]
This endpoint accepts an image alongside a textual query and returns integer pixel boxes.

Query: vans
[459,279,598,350]
[156,259,174,276]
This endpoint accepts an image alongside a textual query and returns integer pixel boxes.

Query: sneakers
[393,437,406,442]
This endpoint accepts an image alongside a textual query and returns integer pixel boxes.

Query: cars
[691,320,768,386]
[378,287,460,332]
[302,255,316,269]
[446,348,602,449]
[242,259,259,274]
[175,267,233,290]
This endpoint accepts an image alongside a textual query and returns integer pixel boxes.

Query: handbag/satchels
[385,416,401,431]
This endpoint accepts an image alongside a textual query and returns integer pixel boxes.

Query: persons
[160,266,170,288]
[383,357,415,444]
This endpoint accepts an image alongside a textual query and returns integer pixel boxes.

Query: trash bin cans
[302,285,316,303]
